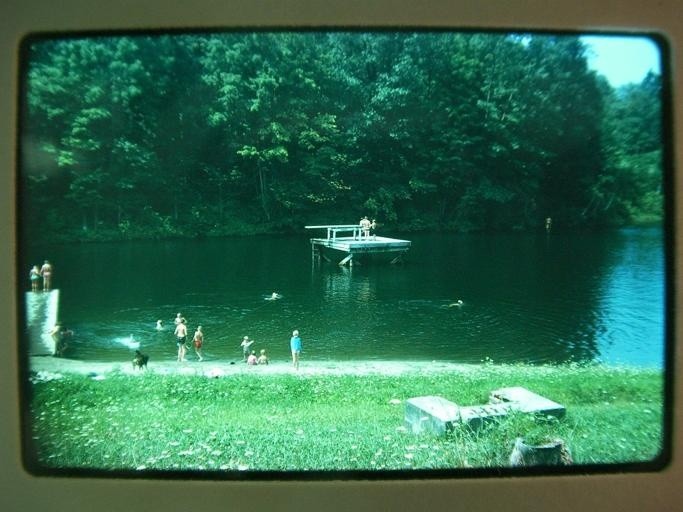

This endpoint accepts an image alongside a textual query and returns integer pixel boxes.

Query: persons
[155,319,164,329]
[174,312,187,325]
[190,325,203,362]
[371,219,378,240]
[173,318,188,362]
[359,215,371,239]
[39,259,52,292]
[240,335,254,359]
[288,329,301,370]
[28,263,40,289]
[246,350,257,366]
[543,214,552,229]
[257,348,269,365]
[60,327,75,335]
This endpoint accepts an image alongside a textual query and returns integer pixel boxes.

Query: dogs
[132,350,149,370]
